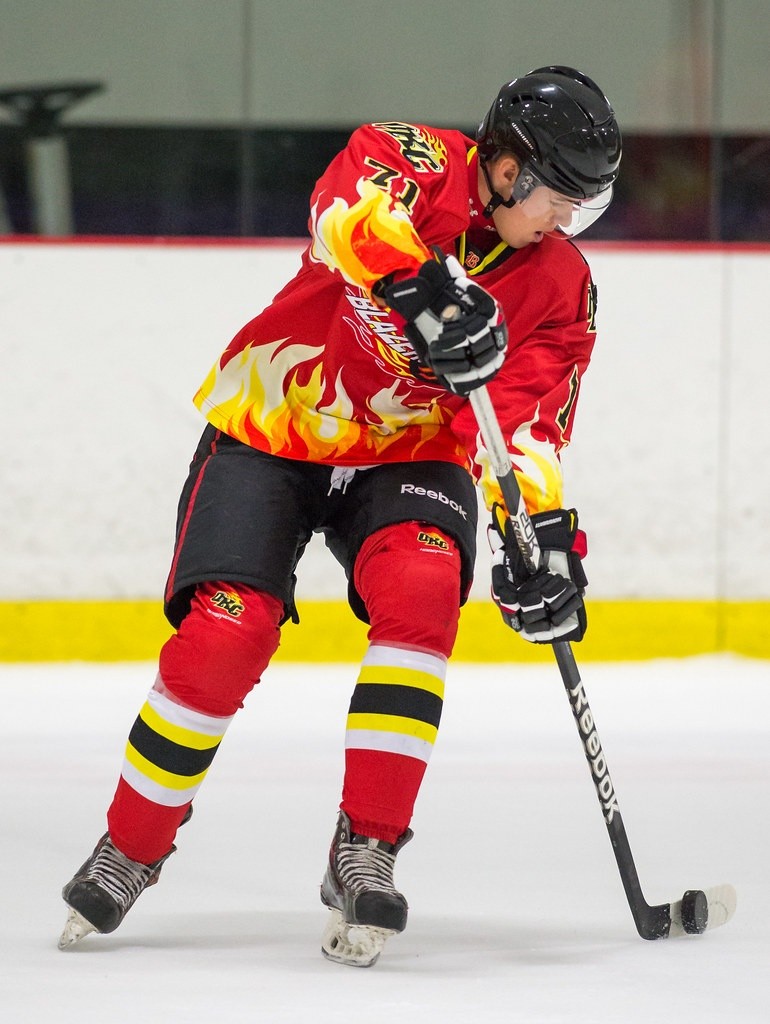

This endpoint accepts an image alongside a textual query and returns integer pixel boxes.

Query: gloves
[486,502,588,645]
[384,254,508,396]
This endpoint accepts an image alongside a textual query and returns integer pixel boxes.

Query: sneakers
[319,808,414,968]
[57,804,194,950]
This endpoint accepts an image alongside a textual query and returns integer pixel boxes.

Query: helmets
[475,65,622,239]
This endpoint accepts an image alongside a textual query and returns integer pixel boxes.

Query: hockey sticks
[441,303,740,942]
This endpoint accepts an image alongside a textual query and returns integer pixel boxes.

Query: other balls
[681,890,708,935]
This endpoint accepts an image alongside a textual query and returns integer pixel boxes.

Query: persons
[54,61,629,935]
